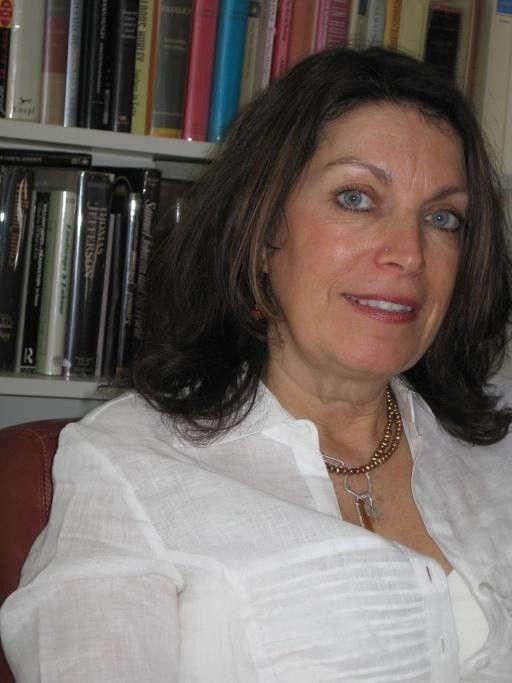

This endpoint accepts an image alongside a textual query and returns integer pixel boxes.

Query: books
[2,1,464,145]
[1,149,197,382]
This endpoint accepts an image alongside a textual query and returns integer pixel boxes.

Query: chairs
[1,416,83,682]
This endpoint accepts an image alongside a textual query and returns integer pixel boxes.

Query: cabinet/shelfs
[0,0,512,434]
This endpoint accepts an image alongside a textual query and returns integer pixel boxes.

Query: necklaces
[264,426,380,534]
[325,384,403,476]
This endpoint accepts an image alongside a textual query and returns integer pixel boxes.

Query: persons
[1,43,512,683]
